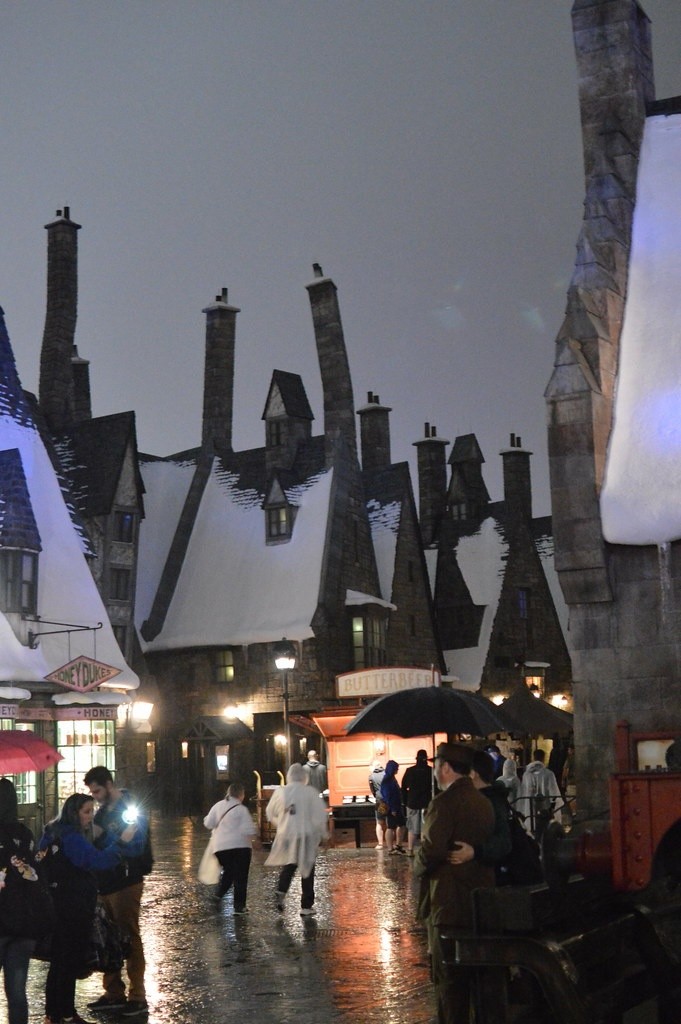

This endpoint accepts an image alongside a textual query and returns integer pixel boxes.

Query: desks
[328,800,376,848]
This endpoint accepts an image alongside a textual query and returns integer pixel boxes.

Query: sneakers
[86,994,127,1010]
[122,999,149,1016]
[44,1010,98,1024]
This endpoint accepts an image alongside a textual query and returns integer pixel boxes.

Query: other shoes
[300,908,316,915]
[387,845,406,857]
[374,844,383,850]
[274,891,285,912]
[407,849,416,858]
[233,908,250,915]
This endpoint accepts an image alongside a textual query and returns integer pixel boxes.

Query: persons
[401,749,439,857]
[202,784,259,916]
[415,740,576,1024]
[380,760,407,856]
[368,761,386,850]
[37,791,139,1024]
[265,763,331,917]
[83,765,150,1016]
[0,777,60,1024]
[303,750,327,792]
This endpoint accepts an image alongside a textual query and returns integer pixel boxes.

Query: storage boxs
[334,819,376,849]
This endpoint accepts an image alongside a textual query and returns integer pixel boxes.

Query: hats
[425,741,472,767]
[470,751,496,778]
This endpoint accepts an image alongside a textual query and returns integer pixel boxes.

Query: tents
[496,676,574,740]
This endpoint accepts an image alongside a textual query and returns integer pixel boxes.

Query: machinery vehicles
[440,718,681,1024]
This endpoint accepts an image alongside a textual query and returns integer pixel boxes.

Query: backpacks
[0,825,58,945]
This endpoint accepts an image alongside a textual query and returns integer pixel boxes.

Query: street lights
[273,636,299,772]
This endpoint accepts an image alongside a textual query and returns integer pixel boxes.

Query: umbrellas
[0,729,66,773]
[341,663,526,799]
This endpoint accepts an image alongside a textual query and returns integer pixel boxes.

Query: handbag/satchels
[199,829,221,885]
[377,801,390,816]
